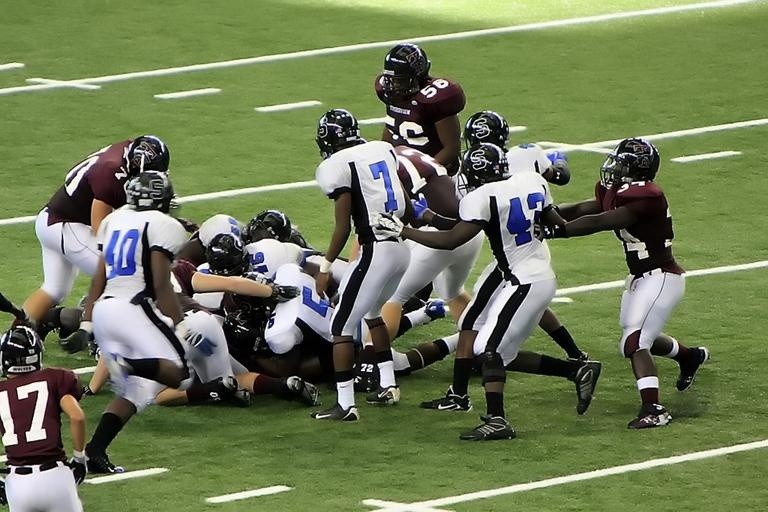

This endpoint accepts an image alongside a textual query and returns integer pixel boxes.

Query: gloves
[370,211,403,237]
[410,192,430,222]
[534,221,568,242]
[182,328,216,356]
[68,452,89,486]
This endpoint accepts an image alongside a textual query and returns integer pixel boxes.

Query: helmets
[600,138,660,191]
[1,325,46,374]
[124,135,170,176]
[315,110,367,159]
[457,144,507,197]
[127,169,173,211]
[462,111,509,151]
[197,209,307,331]
[384,44,431,105]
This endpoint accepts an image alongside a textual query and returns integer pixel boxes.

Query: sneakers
[677,347,709,392]
[627,403,672,429]
[79,445,124,473]
[57,330,90,356]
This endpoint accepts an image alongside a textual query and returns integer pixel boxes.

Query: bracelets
[73,449,85,460]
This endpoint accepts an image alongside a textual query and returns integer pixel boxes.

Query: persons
[535,137,709,429]
[309,110,413,421]
[373,41,463,170]
[377,144,483,361]
[371,144,603,441]
[405,110,587,413]
[0,324,90,512]
[83,208,462,416]
[17,135,169,335]
[72,171,217,472]
